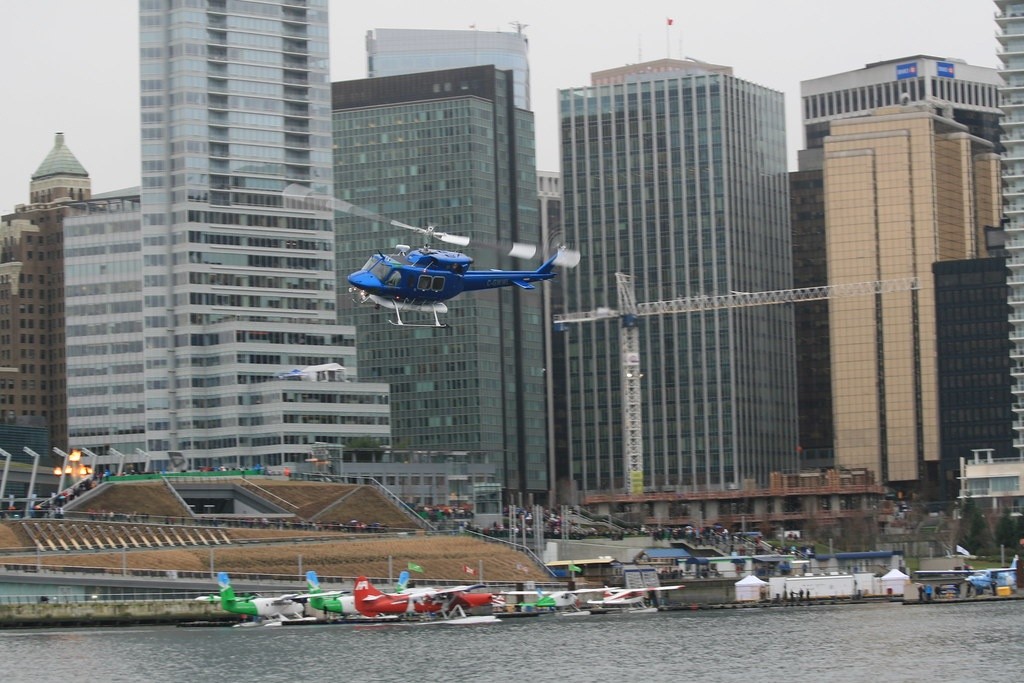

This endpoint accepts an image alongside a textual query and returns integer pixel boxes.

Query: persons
[916,578,998,599]
[0,463,818,556]
[773,589,812,598]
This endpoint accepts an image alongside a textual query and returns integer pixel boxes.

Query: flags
[465,564,475,573]
[516,563,529,570]
[569,562,582,572]
[407,560,424,573]
[956,544,971,556]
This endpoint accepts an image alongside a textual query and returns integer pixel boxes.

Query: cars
[938,584,959,599]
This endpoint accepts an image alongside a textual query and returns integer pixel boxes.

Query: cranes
[551,270,934,515]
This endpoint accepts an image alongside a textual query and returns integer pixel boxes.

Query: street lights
[107,447,127,475]
[81,445,97,480]
[22,445,41,520]
[1,448,12,500]
[51,446,70,498]
[134,448,152,473]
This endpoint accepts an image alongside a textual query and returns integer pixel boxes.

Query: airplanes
[352,574,500,622]
[294,568,410,620]
[901,567,1023,604]
[585,585,685,615]
[281,184,584,332]
[192,571,307,627]
[502,585,621,618]
